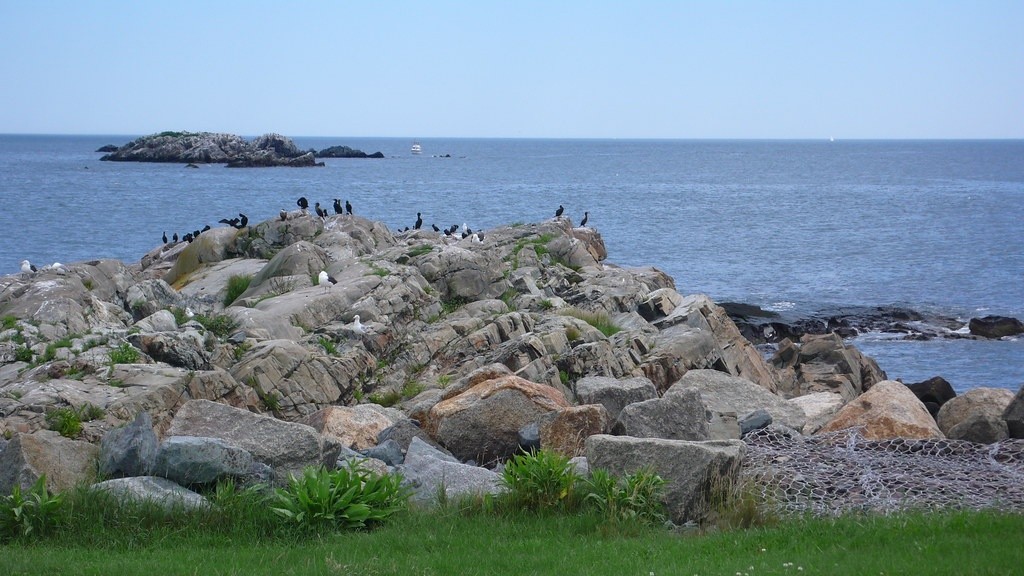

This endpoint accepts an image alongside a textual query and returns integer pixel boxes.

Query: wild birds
[193,230,201,238]
[218,214,248,229]
[319,270,338,293]
[21,260,37,274]
[556,205,564,216]
[444,222,485,242]
[183,233,194,243]
[352,315,374,337]
[173,233,178,242]
[413,212,423,229]
[84,260,100,266]
[332,198,343,214]
[579,212,589,227]
[201,225,210,234]
[345,200,353,215]
[162,231,168,243]
[432,224,440,232]
[280,208,288,221]
[315,202,328,221]
[297,197,308,209]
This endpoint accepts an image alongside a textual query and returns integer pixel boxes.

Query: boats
[411,141,423,155]
